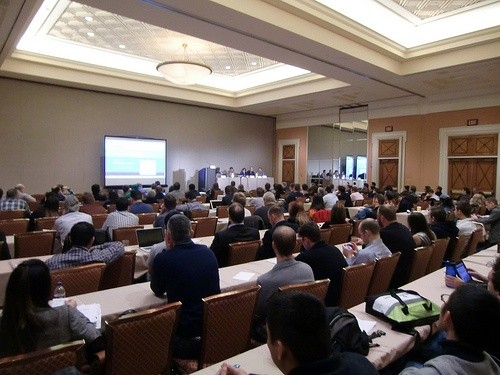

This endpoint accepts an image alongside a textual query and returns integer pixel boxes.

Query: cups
[351,236,358,242]
[122,240,129,246]
[417,206,421,212]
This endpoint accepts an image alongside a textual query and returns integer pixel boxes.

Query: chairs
[0,211,485,375]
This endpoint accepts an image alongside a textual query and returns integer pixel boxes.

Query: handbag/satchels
[329,311,370,356]
[365,287,439,347]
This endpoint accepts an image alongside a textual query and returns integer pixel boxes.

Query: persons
[0,259,103,351]
[343,218,394,265]
[376,203,414,284]
[276,170,500,251]
[220,290,380,375]
[105,181,299,260]
[149,214,220,342]
[0,183,105,245]
[293,222,349,303]
[43,223,124,270]
[399,256,500,375]
[216,167,267,176]
[248,225,315,342]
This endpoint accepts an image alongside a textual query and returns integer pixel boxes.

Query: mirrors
[308,120,368,189]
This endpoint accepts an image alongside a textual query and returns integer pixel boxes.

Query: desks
[49,244,362,326]
[324,178,365,188]
[0,225,262,304]
[396,208,430,227]
[191,243,500,375]
[217,178,274,191]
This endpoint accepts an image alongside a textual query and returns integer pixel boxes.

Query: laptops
[453,258,488,289]
[136,227,165,253]
[210,199,223,209]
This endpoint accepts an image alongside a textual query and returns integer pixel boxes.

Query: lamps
[156,43,212,85]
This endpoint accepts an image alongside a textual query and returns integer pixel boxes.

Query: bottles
[53,282,65,307]
[443,260,457,287]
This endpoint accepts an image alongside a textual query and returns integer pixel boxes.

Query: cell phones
[344,244,354,250]
